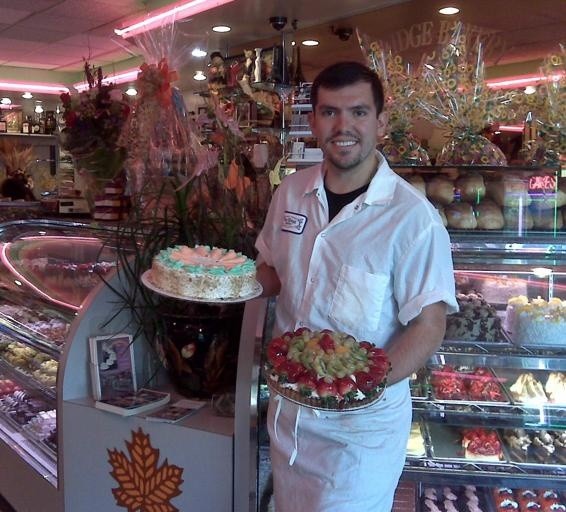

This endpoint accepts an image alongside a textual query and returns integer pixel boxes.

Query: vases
[92,177,125,220]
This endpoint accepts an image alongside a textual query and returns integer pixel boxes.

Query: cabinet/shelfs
[0,220,175,512]
[256,168,566,511]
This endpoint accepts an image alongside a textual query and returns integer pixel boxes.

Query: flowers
[54,57,135,197]
[196,61,251,202]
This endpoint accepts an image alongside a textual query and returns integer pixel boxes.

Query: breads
[404,170,566,238]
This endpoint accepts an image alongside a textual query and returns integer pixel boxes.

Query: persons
[204,60,459,511]
[9,168,38,203]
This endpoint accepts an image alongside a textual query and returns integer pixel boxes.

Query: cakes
[408,365,566,407]
[0,258,119,454]
[407,420,425,456]
[418,486,566,512]
[151,242,258,300]
[454,274,529,309]
[455,427,566,465]
[261,326,391,411]
[504,296,566,348]
[444,291,502,344]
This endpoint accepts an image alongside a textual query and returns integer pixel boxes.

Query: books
[85,333,205,424]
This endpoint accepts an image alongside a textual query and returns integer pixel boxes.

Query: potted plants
[92,168,264,397]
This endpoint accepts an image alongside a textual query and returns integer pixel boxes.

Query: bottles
[22,113,55,135]
[0,117,7,133]
